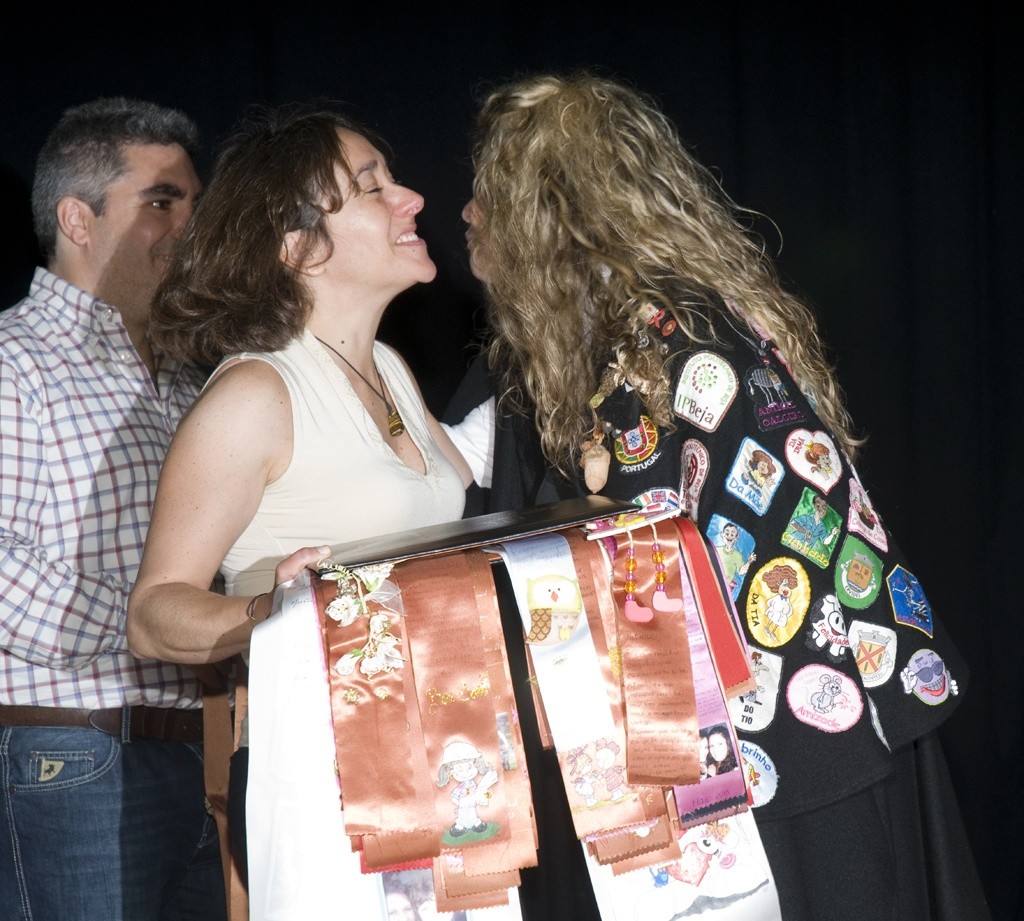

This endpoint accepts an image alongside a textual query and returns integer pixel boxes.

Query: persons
[129,108,534,921]
[0,100,235,921]
[433,71,980,921]
[698,726,737,779]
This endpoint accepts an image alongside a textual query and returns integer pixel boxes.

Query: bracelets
[245,591,272,624]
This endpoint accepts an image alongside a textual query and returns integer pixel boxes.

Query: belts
[0,705,204,741]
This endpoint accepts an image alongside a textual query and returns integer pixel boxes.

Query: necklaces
[314,335,404,437]
